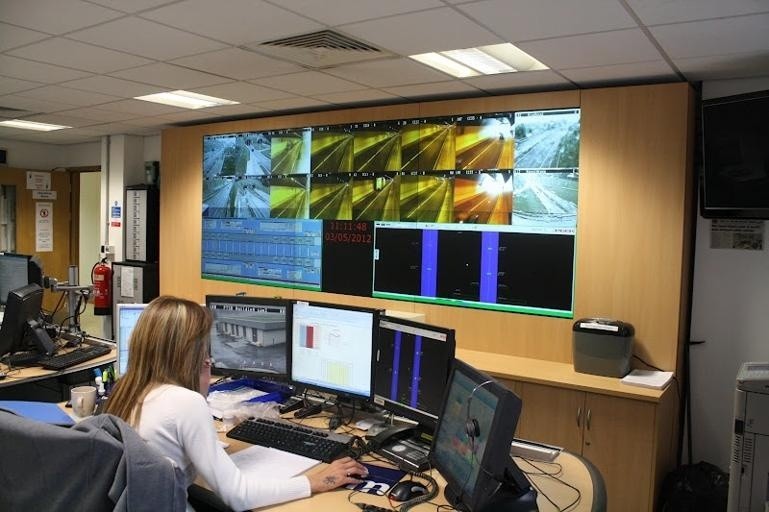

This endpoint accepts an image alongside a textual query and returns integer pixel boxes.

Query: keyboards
[42,344,112,370]
[226,416,355,464]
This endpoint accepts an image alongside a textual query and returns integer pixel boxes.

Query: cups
[70,386,97,417]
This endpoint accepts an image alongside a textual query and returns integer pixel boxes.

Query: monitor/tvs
[288,299,380,431]
[115,303,206,379]
[206,295,289,382]
[0,282,57,368]
[427,358,540,512]
[372,315,456,436]
[0,252,43,306]
[699,89,769,220]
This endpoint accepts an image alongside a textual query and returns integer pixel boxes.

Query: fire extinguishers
[91,257,112,315]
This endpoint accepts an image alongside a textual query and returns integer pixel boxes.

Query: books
[620,368,674,390]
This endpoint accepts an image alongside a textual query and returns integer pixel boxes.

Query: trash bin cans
[661,462,727,512]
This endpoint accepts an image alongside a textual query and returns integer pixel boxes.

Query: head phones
[465,381,498,438]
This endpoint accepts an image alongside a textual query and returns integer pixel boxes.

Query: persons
[97,293,371,511]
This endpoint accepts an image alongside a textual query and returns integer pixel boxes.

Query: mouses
[388,480,429,501]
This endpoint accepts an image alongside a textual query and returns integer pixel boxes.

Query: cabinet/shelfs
[490,375,677,511]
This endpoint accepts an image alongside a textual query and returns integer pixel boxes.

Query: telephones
[369,423,435,472]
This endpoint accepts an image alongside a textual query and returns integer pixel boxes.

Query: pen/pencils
[93,365,115,397]
